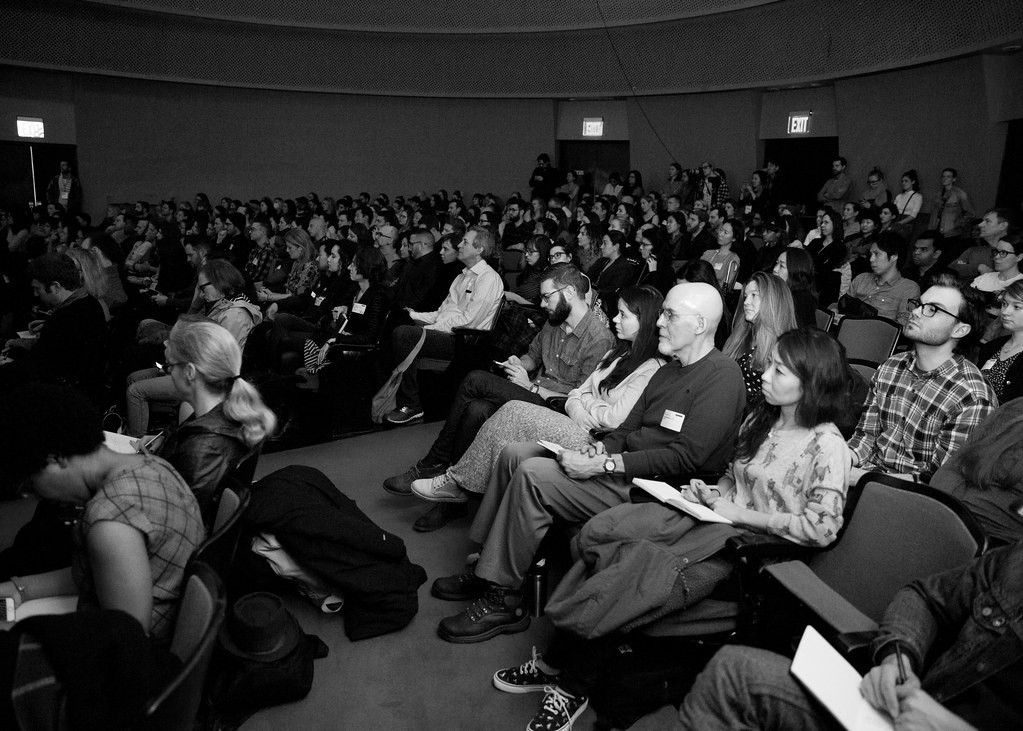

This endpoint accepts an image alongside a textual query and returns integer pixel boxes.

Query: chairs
[116,307,990,731]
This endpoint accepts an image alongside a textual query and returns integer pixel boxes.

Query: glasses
[991,248,1017,258]
[539,285,575,303]
[906,299,967,323]
[867,178,882,186]
[376,231,391,239]
[163,360,189,374]
[199,280,213,293]
[639,241,653,248]
[547,252,568,261]
[524,248,540,254]
[408,241,427,247]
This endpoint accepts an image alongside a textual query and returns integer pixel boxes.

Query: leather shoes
[272,418,300,439]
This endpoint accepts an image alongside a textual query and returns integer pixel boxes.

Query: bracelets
[530,383,539,393]
[710,488,721,497]
[267,294,271,301]
[11,577,31,601]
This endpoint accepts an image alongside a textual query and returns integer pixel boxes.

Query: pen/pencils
[893,641,907,681]
[680,485,720,490]
[3,350,10,360]
[141,428,166,452]
[492,359,505,366]
[330,308,338,312]
[588,442,608,455]
[37,310,53,316]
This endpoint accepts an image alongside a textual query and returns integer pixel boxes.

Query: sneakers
[383,459,447,496]
[414,503,469,531]
[432,558,497,601]
[383,406,424,424]
[411,472,469,503]
[525,686,589,731]
[493,645,560,693]
[437,586,531,644]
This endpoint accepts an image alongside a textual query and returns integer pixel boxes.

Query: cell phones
[492,360,505,366]
[0,597,16,622]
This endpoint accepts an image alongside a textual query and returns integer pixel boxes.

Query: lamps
[16,117,44,138]
[582,117,605,137]
[787,109,813,135]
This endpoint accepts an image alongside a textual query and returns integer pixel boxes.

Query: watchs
[604,453,616,473]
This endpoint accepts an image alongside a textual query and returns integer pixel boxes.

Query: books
[790,625,894,731]
[633,479,733,524]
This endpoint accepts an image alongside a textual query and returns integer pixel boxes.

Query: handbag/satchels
[371,375,402,424]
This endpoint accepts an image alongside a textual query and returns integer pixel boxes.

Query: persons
[0,153,1023,731]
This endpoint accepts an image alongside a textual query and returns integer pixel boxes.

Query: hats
[228,212,246,233]
[219,592,300,663]
